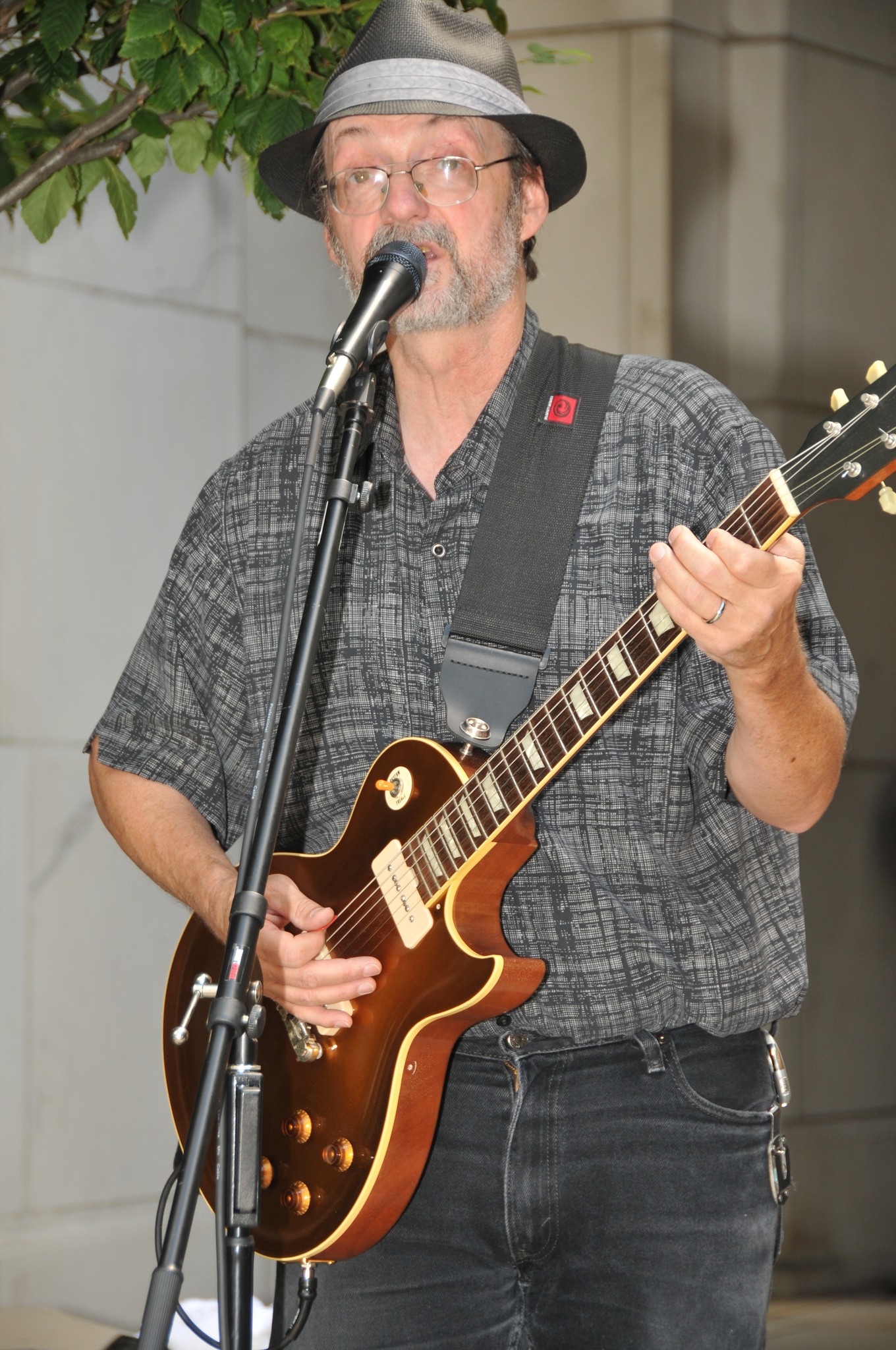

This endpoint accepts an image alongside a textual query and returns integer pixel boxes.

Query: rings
[702,598,726,624]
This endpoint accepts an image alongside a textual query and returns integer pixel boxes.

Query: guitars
[160,354,895,1269]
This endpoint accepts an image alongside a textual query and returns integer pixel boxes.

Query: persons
[81,4,858,1349]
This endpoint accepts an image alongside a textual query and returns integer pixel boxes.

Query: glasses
[315,154,524,217]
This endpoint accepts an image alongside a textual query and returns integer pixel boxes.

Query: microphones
[312,241,428,418]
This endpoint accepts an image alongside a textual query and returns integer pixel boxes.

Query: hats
[259,1,589,222]
